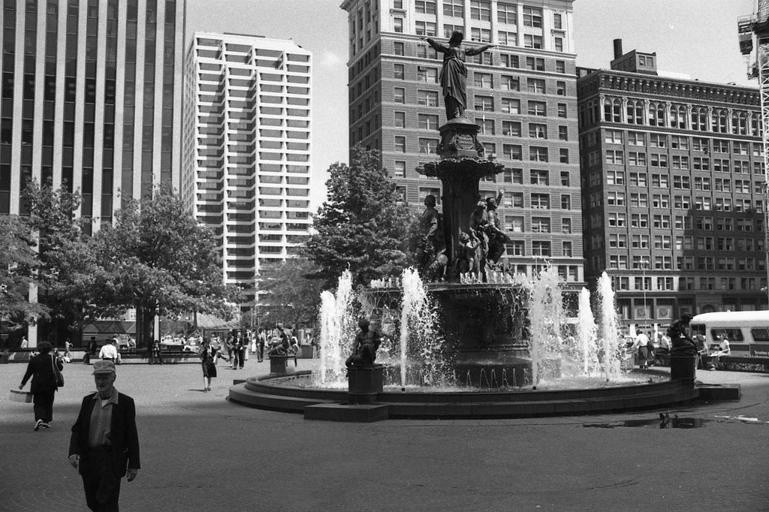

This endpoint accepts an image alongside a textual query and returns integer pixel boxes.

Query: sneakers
[33,419,51,431]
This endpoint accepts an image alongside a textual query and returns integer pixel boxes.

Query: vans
[529,318,596,354]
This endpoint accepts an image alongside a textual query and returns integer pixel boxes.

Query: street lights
[639,260,650,339]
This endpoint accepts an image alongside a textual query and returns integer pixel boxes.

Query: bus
[688,309,769,372]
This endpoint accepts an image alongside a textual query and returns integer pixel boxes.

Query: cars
[620,336,672,368]
[113,330,204,354]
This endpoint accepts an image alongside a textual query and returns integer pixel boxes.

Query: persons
[152,340,163,364]
[345,317,381,378]
[267,324,300,357]
[127,338,135,347]
[226,329,265,369]
[20,336,27,348]
[68,360,141,512]
[418,29,497,119]
[54,338,73,363]
[100,334,121,363]
[83,337,97,364]
[620,329,731,369]
[667,313,696,347]
[200,338,223,391]
[19,342,63,430]
[413,189,511,281]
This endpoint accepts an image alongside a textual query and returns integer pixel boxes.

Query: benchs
[701,341,769,373]
[87,341,200,358]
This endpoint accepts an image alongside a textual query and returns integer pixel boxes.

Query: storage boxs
[9,389,32,403]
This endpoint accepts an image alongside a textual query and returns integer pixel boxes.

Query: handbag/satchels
[52,368,64,387]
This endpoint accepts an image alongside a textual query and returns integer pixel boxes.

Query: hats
[94,360,116,374]
